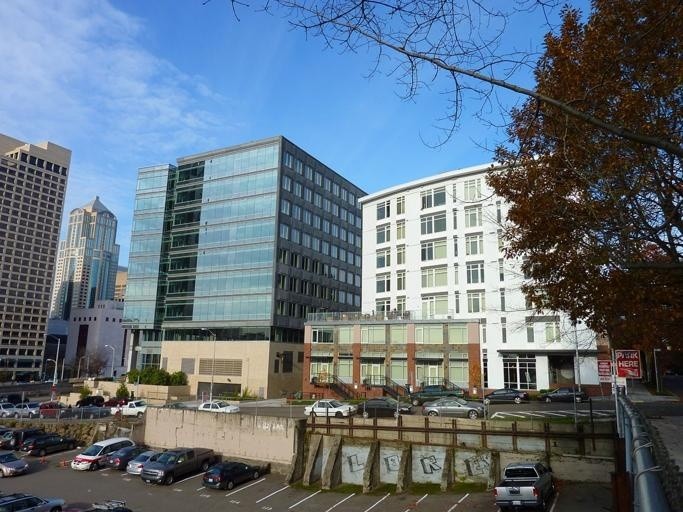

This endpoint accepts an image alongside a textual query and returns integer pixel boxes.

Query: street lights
[44,332,61,400]
[105,345,115,379]
[77,352,95,377]
[201,328,217,400]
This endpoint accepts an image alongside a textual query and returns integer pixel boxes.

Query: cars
[304,399,357,418]
[357,398,412,418]
[201,463,260,490]
[485,388,528,404]
[422,398,487,419]
[0,394,239,419]
[0,492,68,512]
[71,437,214,485]
[0,424,76,477]
[537,387,589,403]
[78,500,133,512]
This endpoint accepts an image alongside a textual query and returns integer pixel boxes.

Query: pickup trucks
[411,386,467,406]
[494,463,555,512]
